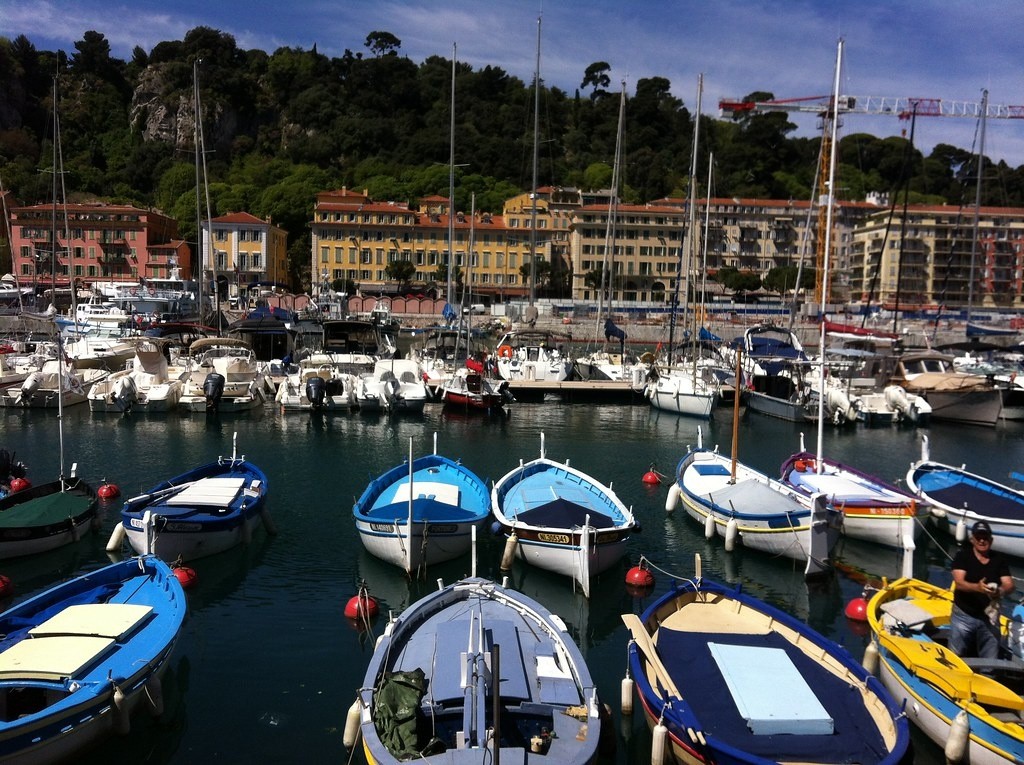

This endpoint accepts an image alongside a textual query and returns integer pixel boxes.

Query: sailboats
[778,35,947,552]
[0,76,98,562]
[0,11,1024,432]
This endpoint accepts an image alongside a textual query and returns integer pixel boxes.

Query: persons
[949,517,1015,679]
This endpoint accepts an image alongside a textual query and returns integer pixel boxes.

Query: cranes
[715,96,1024,320]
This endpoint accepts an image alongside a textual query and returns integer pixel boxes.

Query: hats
[972,520,992,534]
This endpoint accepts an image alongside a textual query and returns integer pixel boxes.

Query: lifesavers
[498,344,512,359]
[793,459,823,472]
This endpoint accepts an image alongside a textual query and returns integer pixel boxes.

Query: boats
[354,523,603,765]
[352,432,489,577]
[0,509,189,765]
[905,433,1024,558]
[675,341,844,573]
[866,574,1024,765]
[489,428,640,598]
[620,553,909,765]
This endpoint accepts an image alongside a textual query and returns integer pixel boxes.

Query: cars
[120,431,270,565]
